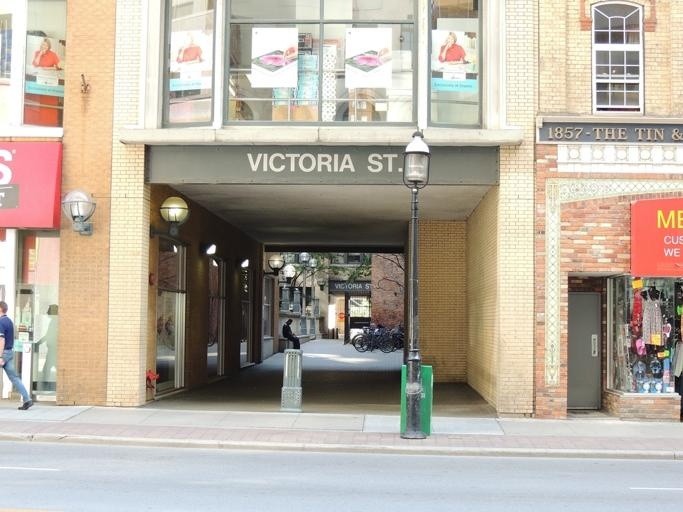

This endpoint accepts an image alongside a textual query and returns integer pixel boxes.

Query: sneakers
[17,401,33,410]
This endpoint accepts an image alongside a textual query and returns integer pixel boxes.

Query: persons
[282,319,300,349]
[32,38,63,70]
[0,301,33,410]
[439,32,466,65]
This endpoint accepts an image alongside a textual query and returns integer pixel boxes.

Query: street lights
[300,251,318,335]
[402,130,431,439]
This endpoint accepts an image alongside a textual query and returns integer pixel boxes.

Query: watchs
[0,357,4,358]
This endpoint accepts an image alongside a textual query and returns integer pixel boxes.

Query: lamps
[150,196,190,238]
[61,190,97,235]
[262,254,285,276]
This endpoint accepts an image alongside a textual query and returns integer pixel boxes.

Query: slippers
[635,339,647,356]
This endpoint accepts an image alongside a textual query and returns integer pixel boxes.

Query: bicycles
[352,324,404,353]
